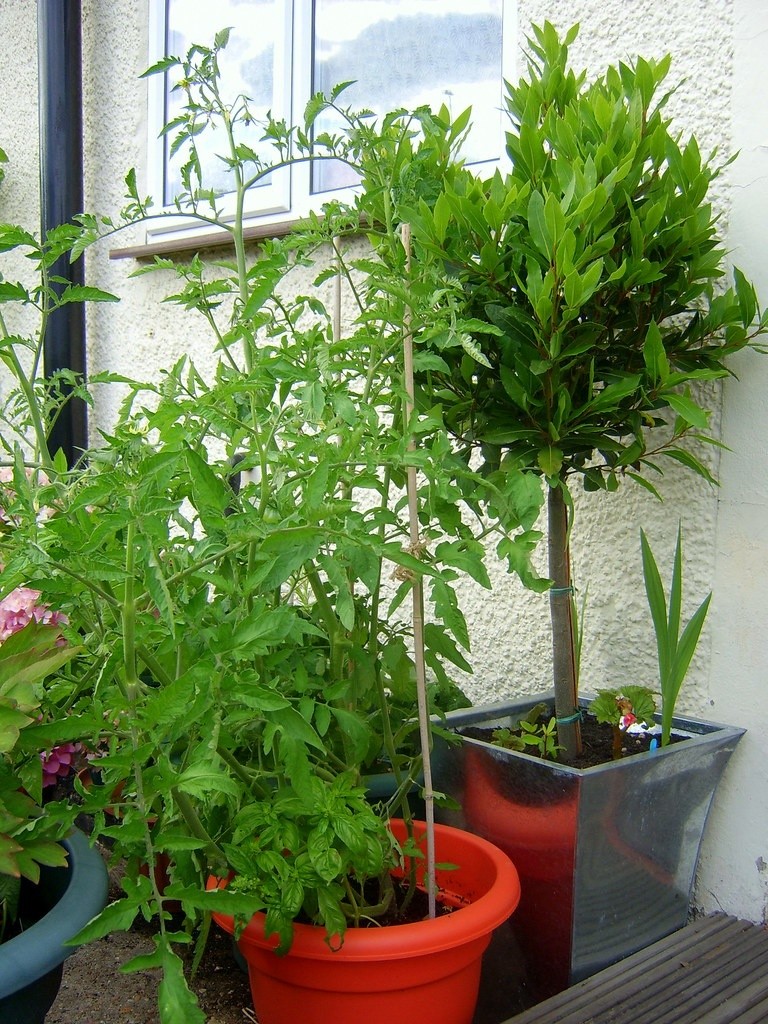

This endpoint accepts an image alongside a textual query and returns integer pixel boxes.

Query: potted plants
[359,15,768,1005]
[2,208,519,1024]
